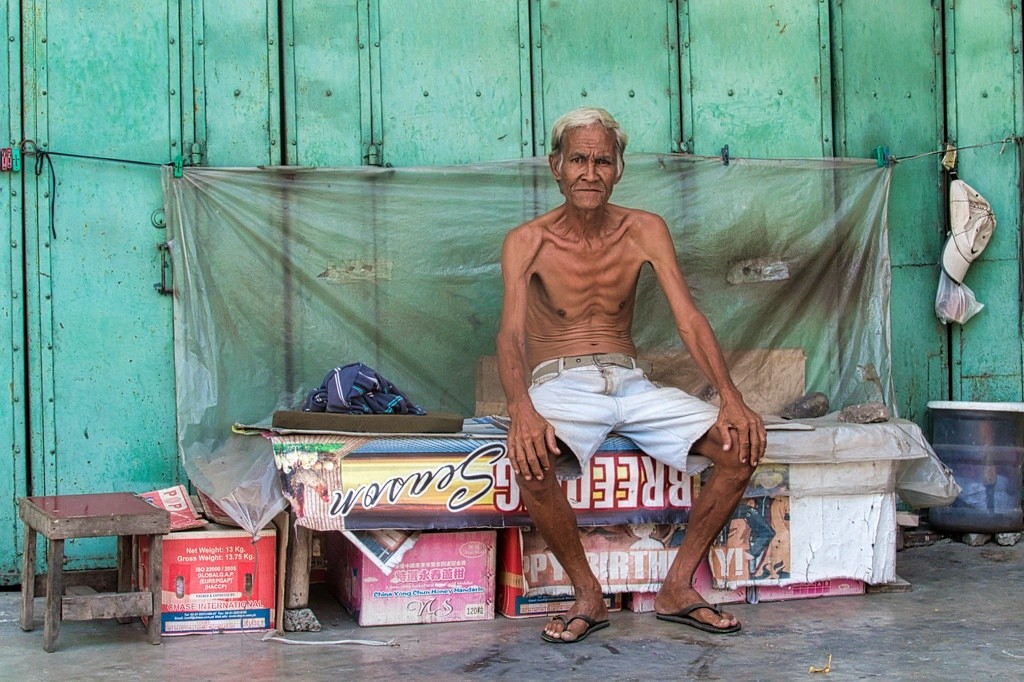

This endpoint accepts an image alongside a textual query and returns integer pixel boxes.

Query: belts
[531,352,653,382]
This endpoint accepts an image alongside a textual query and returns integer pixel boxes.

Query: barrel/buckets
[924,401,1024,532]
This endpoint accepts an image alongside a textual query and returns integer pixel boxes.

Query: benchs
[234,411,924,633]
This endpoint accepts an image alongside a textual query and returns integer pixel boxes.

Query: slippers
[540,613,611,644]
[652,603,742,635]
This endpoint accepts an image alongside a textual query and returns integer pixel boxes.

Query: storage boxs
[130,485,277,636]
[310,524,866,627]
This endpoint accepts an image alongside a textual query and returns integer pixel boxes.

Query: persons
[495,107,768,644]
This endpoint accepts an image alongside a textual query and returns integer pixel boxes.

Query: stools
[19,492,169,654]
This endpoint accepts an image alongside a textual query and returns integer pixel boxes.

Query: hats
[942,174,996,286]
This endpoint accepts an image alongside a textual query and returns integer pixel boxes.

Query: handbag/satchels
[935,263,986,326]
